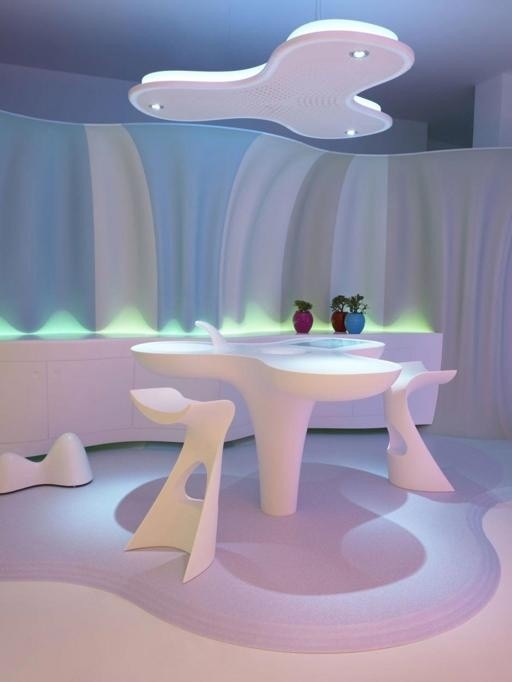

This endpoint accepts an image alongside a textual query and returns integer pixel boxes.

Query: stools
[124,388,236,582]
[385,358,458,493]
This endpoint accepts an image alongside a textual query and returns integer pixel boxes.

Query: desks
[131,321,402,516]
[0,333,444,456]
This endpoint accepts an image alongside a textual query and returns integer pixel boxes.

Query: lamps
[127,0,415,140]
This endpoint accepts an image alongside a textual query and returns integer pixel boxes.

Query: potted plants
[291,299,314,335]
[345,294,368,334]
[329,295,349,334]
